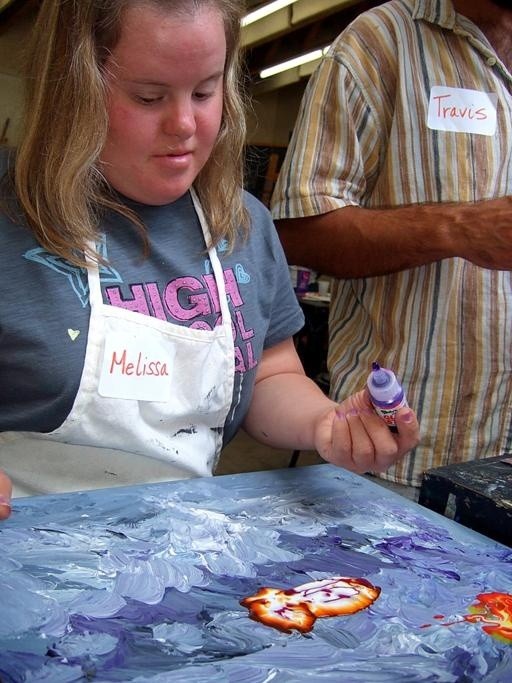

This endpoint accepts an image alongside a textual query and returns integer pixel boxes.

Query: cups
[318,280,330,296]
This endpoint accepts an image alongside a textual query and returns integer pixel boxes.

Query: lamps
[257,42,331,79]
[238,0,298,28]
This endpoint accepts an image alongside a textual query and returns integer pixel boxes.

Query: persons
[266,0,512,520]
[0,0,423,522]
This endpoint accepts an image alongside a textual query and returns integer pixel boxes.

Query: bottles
[366,362,410,434]
